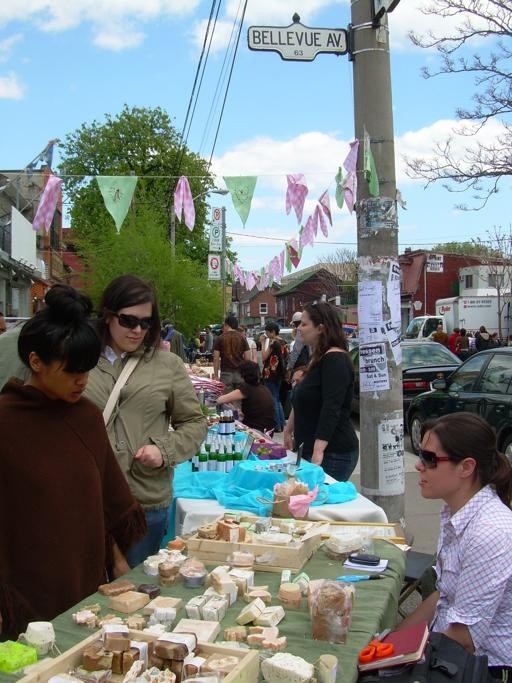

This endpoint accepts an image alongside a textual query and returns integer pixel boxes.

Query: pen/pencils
[338,574,388,583]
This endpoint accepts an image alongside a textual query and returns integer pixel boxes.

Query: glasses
[105,309,154,329]
[418,451,473,469]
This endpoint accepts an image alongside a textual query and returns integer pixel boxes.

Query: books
[356,623,430,673]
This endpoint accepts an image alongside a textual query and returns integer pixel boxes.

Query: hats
[290,312,302,325]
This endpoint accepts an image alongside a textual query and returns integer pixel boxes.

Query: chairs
[216,402,239,420]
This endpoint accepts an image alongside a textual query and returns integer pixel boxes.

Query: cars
[348,341,463,425]
[407,347,512,466]
[263,329,295,352]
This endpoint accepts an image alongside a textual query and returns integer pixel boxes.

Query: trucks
[406,295,512,345]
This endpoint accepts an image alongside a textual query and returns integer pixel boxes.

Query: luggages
[359,631,487,678]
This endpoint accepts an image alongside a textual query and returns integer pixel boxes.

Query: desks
[182,364,221,378]
[168,417,389,535]
[0,518,414,683]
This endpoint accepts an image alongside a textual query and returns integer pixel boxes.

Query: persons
[81,274,207,569]
[186,313,311,439]
[394,410,512,683]
[1,283,147,642]
[433,324,469,354]
[468,326,500,354]
[284,302,359,482]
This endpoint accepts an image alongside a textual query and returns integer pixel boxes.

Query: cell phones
[350,552,379,564]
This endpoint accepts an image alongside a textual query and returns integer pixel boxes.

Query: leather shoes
[275,427,282,433]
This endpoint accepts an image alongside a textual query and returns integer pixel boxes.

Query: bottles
[198,387,205,407]
[192,435,242,473]
[219,410,235,435]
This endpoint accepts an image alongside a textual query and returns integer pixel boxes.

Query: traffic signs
[210,225,222,250]
[370,0,401,28]
[425,253,444,273]
[212,207,223,222]
[207,254,222,281]
[246,11,348,63]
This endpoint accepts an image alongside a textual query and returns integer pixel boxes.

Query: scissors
[359,626,395,662]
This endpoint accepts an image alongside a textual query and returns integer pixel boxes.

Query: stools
[398,550,437,619]
